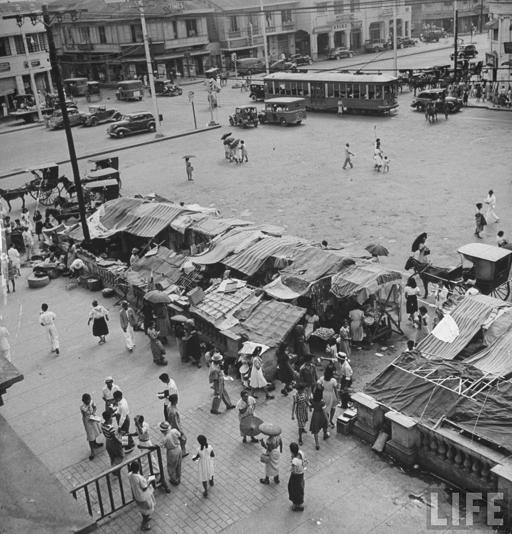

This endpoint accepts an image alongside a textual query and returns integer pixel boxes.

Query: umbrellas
[182,154,197,158]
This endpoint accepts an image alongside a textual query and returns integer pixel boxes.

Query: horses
[426,102,439,125]
[404,255,456,300]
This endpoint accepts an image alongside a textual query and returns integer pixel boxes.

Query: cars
[286,53,313,65]
[229,105,257,128]
[329,46,356,62]
[451,42,478,60]
[391,36,413,49]
[45,74,183,140]
[398,61,474,113]
[9,94,35,111]
[247,84,264,101]
[265,58,297,72]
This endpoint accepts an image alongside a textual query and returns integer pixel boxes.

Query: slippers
[261,479,269,484]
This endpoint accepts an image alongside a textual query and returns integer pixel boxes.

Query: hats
[132,247,140,254]
[240,415,264,436]
[158,420,172,431]
[335,351,348,360]
[258,421,281,435]
[74,259,83,270]
[211,352,223,361]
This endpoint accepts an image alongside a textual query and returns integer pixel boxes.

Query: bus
[263,70,401,117]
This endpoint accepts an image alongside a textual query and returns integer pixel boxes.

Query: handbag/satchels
[260,447,270,463]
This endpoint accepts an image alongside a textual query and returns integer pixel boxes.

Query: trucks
[8,92,78,122]
[235,56,266,74]
[419,27,443,42]
[258,96,307,126]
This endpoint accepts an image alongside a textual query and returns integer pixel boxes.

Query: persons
[208,68,266,107]
[287,442,306,512]
[374,145,382,173]
[220,131,249,163]
[185,157,195,181]
[383,156,391,173]
[337,99,344,117]
[473,202,487,239]
[342,143,355,170]
[398,64,512,127]
[79,230,512,487]
[375,138,381,143]
[192,435,215,497]
[39,303,59,356]
[127,462,156,530]
[484,190,500,224]
[0,327,10,363]
[376,142,384,154]
[1,84,73,120]
[0,191,80,289]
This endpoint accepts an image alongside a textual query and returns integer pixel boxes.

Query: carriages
[404,242,512,301]
[45,152,121,223]
[204,67,230,88]
[0,161,61,212]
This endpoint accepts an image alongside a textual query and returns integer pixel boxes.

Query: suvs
[363,38,389,53]
